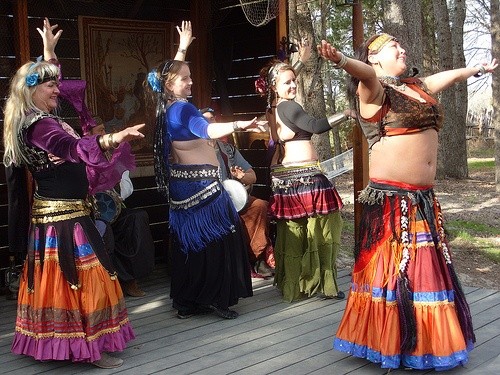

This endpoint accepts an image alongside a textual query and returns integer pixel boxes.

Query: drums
[95,189,122,224]
[222,180,250,214]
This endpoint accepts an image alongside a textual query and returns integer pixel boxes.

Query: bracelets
[232,120,240,131]
[108,132,121,150]
[327,112,348,128]
[177,48,187,54]
[291,60,305,75]
[98,135,109,153]
[333,51,348,70]
[473,64,485,78]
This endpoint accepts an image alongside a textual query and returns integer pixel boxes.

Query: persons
[142,20,268,321]
[261,38,357,304]
[197,108,277,280]
[2,16,145,369]
[317,33,498,371]
[83,115,147,297]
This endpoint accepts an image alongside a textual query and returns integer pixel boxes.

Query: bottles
[5,255,23,301]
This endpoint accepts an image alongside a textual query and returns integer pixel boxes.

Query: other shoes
[89,351,123,369]
[317,292,345,299]
[254,259,272,276]
[125,280,145,296]
[177,307,214,317]
[210,304,238,318]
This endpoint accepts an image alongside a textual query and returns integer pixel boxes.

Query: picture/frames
[77,16,174,167]
